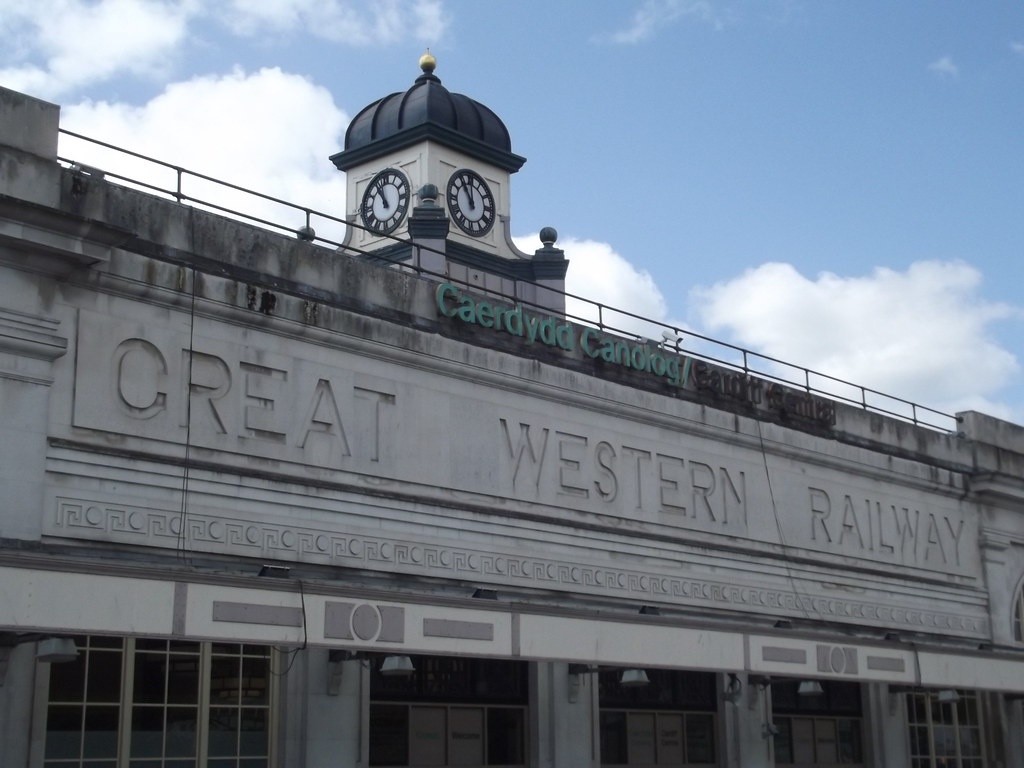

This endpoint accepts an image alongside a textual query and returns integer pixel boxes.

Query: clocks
[448,168,496,238]
[359,168,410,237]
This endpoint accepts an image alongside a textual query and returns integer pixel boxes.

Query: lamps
[380,657,416,677]
[938,690,959,701]
[35,638,80,662]
[798,681,823,694]
[621,669,649,686]
